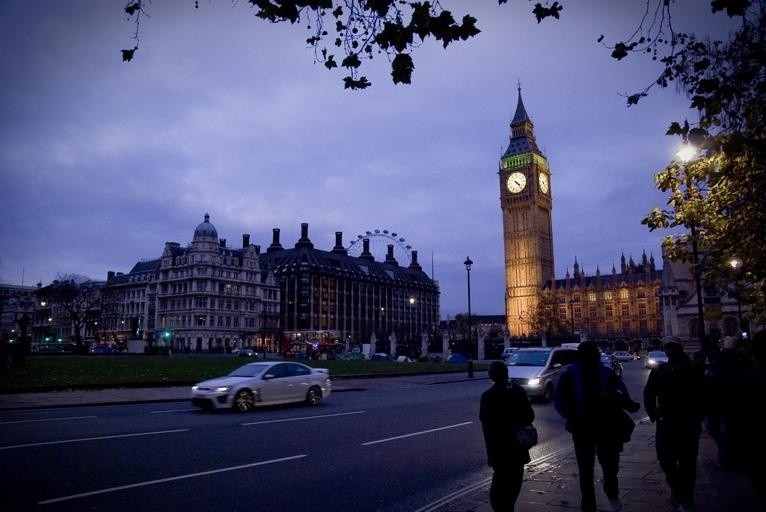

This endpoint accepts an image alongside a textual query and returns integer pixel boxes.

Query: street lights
[677,144,706,340]
[463,255,473,341]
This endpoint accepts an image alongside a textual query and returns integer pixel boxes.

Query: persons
[555,340,641,512]
[478,360,538,512]
[643,336,750,512]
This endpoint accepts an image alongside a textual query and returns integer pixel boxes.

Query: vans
[505,346,578,405]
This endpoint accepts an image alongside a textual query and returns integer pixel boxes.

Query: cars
[600,354,625,378]
[191,360,333,414]
[238,349,258,358]
[645,351,669,369]
[612,351,634,362]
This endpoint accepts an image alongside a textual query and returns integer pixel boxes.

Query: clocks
[506,171,528,194]
[538,172,549,195]
